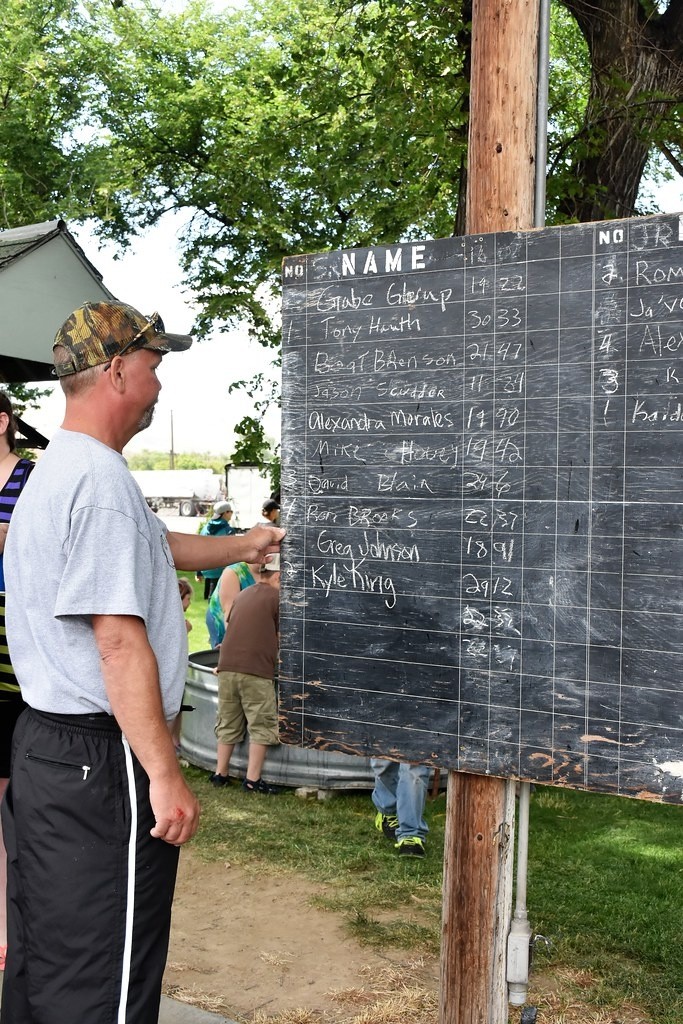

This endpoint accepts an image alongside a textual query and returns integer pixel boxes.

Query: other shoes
[0,945,8,971]
[177,757,191,769]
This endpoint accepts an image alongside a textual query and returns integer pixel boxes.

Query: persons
[369,757,432,859]
[173,580,194,749]
[0,394,34,974]
[209,553,280,794]
[0,300,287,1024]
[254,500,282,529]
[195,501,236,599]
[205,561,263,649]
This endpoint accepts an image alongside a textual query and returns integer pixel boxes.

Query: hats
[261,500,281,513]
[259,551,280,573]
[210,500,232,520]
[52,302,194,378]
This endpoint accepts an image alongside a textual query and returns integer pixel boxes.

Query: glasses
[227,510,234,514]
[104,313,166,373]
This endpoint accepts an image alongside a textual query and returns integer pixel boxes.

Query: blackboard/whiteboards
[278,213,682,808]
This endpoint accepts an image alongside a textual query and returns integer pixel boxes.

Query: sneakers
[374,810,402,841]
[394,836,427,859]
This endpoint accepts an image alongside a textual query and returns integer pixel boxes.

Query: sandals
[241,776,279,795]
[208,771,231,787]
